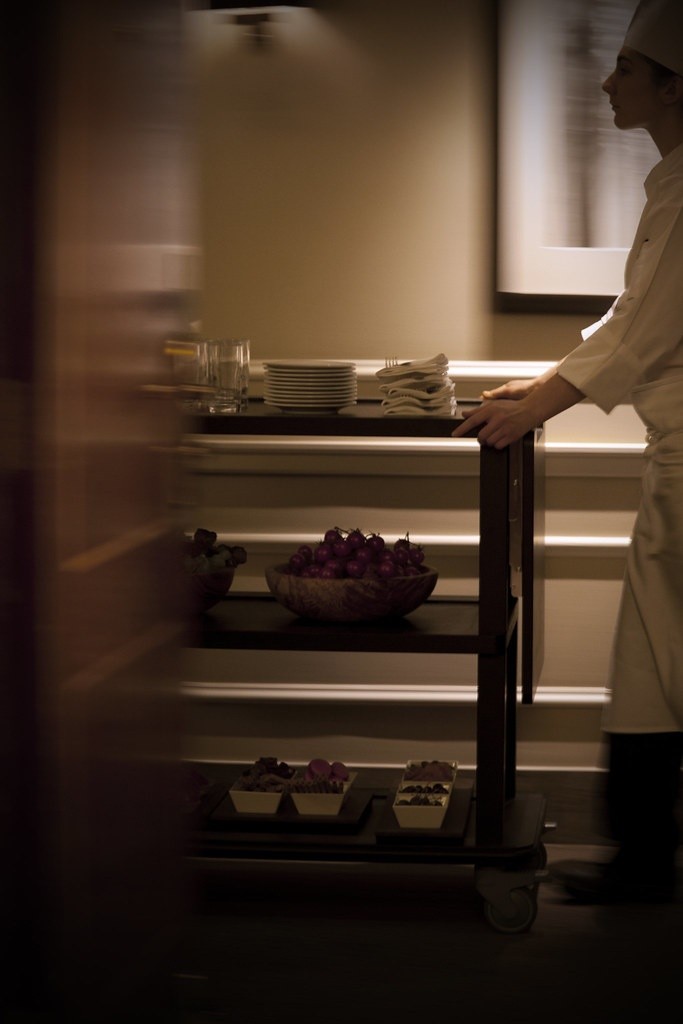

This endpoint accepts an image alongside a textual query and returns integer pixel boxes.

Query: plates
[392,759,458,828]
[262,360,359,415]
[228,768,358,815]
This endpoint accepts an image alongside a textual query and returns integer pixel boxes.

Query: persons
[441,1,683,906]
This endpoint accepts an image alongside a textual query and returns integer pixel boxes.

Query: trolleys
[179,399,549,936]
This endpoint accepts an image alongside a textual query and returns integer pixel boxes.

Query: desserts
[232,755,351,795]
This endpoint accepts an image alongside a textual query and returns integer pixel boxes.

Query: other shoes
[566,870,677,906]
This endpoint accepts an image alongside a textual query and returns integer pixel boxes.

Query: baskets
[183,568,236,612]
[265,562,439,621]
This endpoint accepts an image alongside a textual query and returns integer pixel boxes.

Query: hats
[623,0,683,76]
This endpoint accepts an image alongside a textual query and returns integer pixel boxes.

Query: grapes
[184,543,246,574]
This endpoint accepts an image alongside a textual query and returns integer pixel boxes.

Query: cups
[173,336,249,414]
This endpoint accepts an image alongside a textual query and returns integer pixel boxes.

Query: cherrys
[282,526,426,579]
